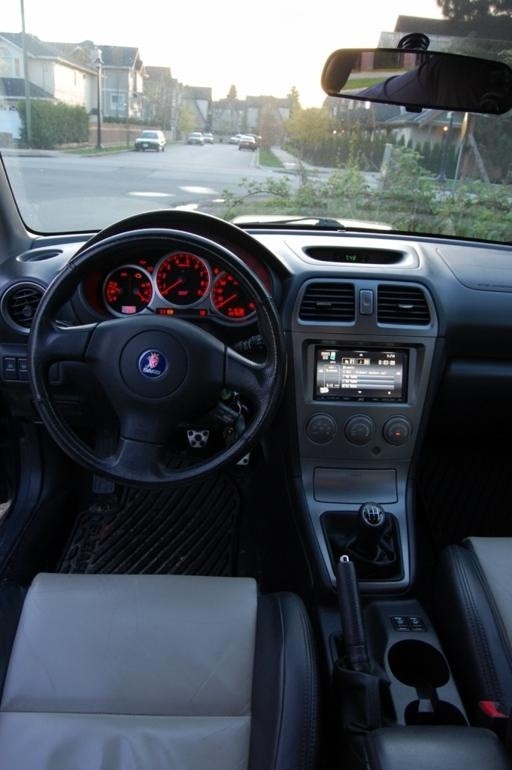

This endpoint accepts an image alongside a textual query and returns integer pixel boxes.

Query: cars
[185,132,261,151]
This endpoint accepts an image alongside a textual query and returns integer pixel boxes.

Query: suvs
[134,129,167,152]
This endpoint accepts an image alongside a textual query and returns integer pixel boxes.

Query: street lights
[437,125,449,178]
[93,58,106,150]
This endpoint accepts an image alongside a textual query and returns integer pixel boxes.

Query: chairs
[446,534,511,716]
[0,566,320,769]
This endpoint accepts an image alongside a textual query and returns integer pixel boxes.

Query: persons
[354,54,469,111]
